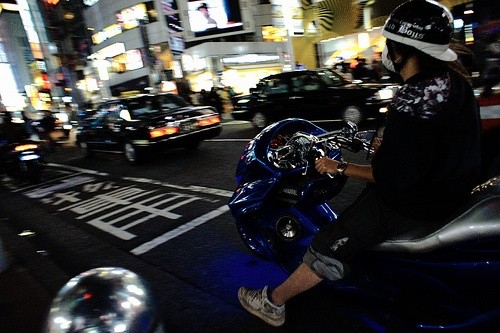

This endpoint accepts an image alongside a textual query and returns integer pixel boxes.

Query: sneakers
[238,285,285,326]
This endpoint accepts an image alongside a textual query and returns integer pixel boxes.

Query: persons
[238,0,483,326]
[199,83,241,117]
[65,103,81,147]
[196,1,218,31]
[0,110,57,148]
[334,54,396,83]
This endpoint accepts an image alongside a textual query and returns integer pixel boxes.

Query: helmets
[382,0,457,61]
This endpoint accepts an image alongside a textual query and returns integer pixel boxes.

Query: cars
[74,93,223,166]
[0,107,99,185]
[229,68,402,133]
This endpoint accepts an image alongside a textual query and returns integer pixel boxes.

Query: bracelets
[338,161,348,176]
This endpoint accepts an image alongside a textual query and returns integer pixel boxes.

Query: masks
[382,43,401,72]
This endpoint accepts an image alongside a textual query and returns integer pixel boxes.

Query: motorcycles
[228,117,500,333]
